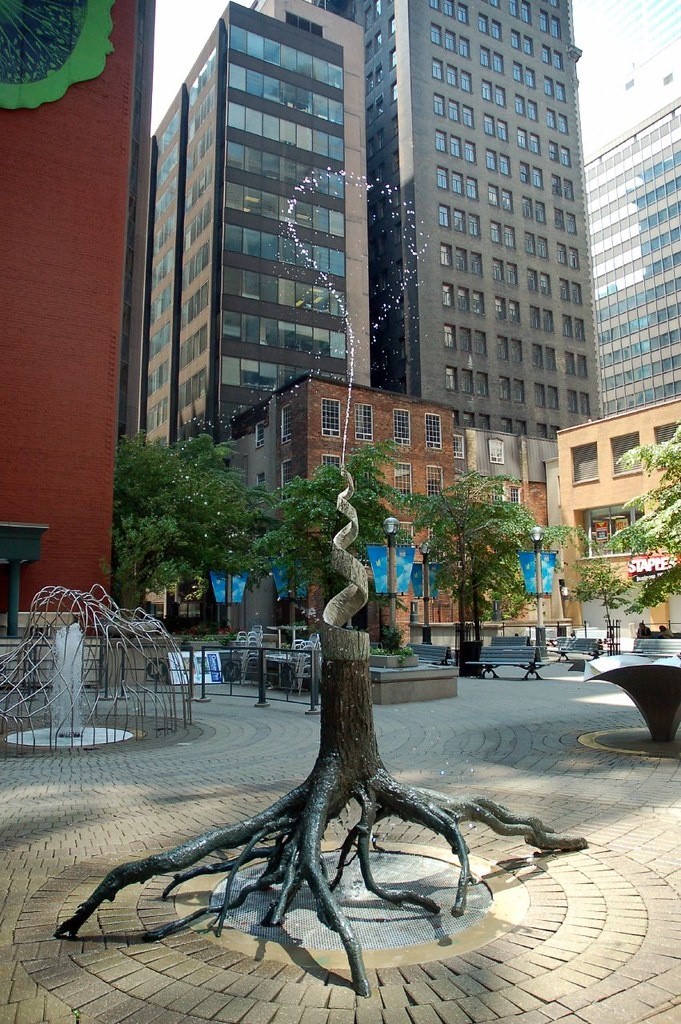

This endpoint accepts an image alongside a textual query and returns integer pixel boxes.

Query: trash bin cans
[460,639,483,676]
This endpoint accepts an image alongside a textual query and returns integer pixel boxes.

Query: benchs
[370,636,681,681]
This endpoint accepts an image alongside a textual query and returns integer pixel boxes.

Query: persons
[654,625,674,639]
[636,623,652,639]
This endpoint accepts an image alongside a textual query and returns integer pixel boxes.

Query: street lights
[418,541,432,645]
[381,516,401,629]
[529,526,548,657]
